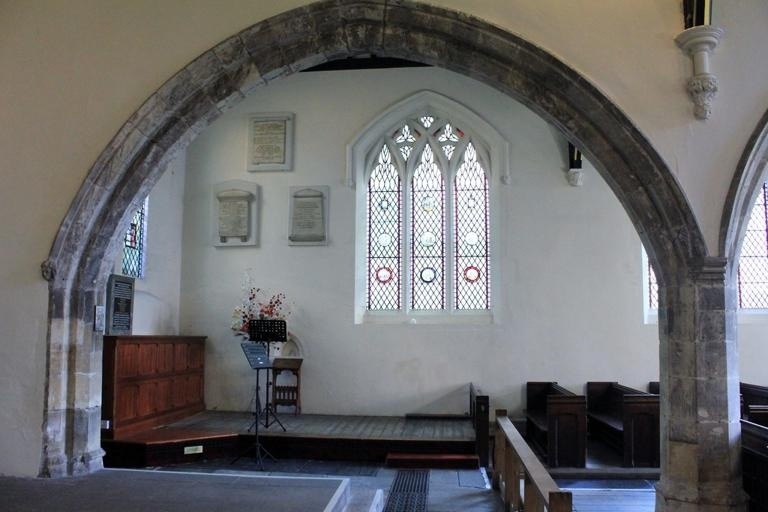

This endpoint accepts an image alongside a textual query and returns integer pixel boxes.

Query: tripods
[247,342,287,433]
[228,368,278,472]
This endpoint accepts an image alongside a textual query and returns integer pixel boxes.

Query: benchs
[733,374,768,509]
[525,376,665,468]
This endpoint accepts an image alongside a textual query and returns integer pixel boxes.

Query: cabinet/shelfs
[100,334,205,440]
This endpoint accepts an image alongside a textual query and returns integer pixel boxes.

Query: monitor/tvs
[105,275,136,335]
